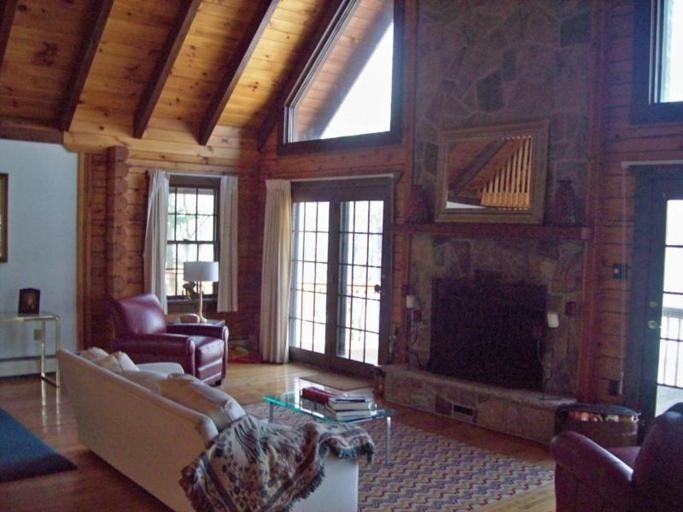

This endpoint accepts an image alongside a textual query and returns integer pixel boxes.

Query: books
[323,390,378,421]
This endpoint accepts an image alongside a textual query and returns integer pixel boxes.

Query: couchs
[108,294,230,388]
[554,401,682,510]
[51,348,361,512]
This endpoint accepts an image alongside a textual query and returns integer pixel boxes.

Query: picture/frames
[434,116,553,225]
[17,287,40,315]
[0,171,8,264]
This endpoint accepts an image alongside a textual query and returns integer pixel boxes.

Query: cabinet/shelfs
[385,373,438,412]
[479,392,556,441]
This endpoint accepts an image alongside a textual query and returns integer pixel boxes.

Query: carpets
[0,405,78,483]
[229,399,557,512]
[297,371,371,393]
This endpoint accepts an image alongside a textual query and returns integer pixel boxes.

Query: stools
[559,400,640,444]
[374,366,386,394]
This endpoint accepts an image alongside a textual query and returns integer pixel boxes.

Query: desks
[0,310,66,388]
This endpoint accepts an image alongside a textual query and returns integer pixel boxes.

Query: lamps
[399,292,424,367]
[182,261,219,321]
[542,309,570,399]
